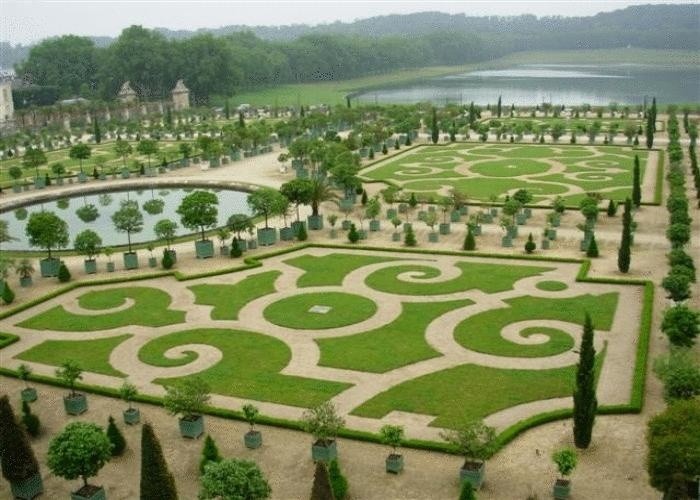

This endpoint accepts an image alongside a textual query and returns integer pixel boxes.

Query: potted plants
[200,459,273,500]
[17,364,39,401]
[297,183,340,231]
[140,424,178,498]
[75,231,102,274]
[120,383,141,426]
[106,417,126,458]
[277,198,310,241]
[3,397,43,498]
[327,458,349,498]
[25,212,70,277]
[46,422,115,500]
[104,248,116,272]
[323,180,598,253]
[218,213,257,258]
[147,243,157,269]
[55,363,88,415]
[179,190,218,258]
[18,259,35,288]
[302,400,345,463]
[243,405,262,448]
[311,460,333,498]
[382,425,403,473]
[552,449,576,499]
[459,477,474,498]
[155,220,177,267]
[199,437,223,475]
[58,264,74,285]
[249,186,286,246]
[442,422,498,492]
[163,377,212,440]
[113,204,143,270]
